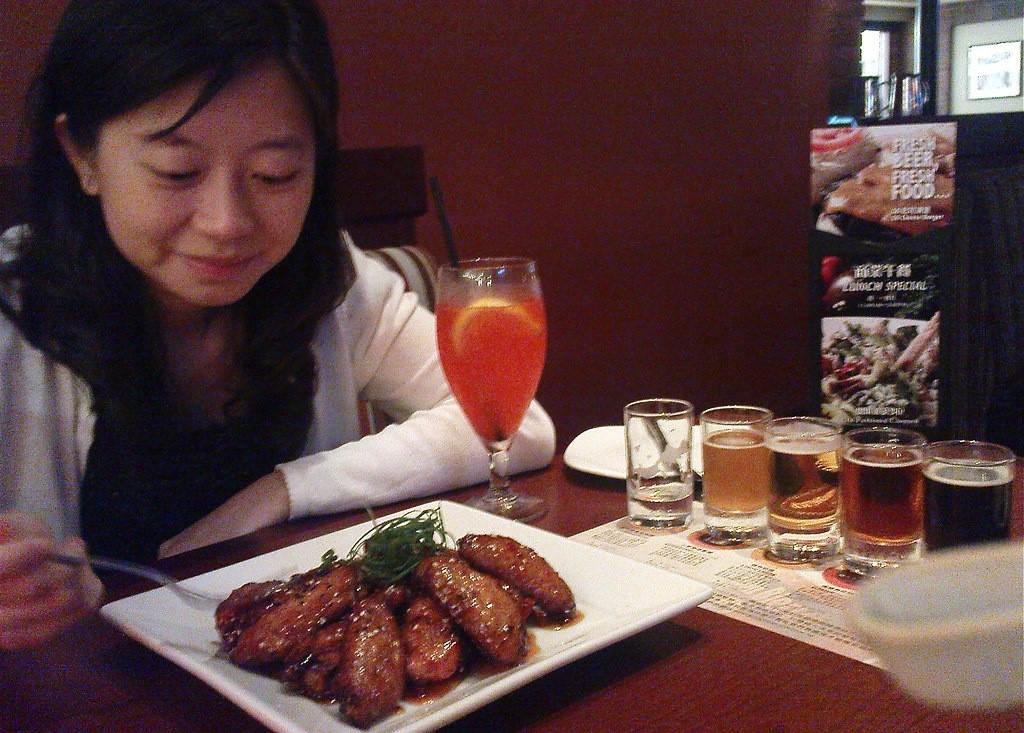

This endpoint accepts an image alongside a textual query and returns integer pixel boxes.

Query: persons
[0,0,557,649]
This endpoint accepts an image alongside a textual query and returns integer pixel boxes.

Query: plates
[98,499,713,733]
[564,423,751,480]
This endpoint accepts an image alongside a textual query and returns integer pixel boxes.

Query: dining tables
[0,449,1024,733]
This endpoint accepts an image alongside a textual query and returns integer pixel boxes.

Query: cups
[622,397,696,533]
[762,416,843,561]
[921,439,1015,553]
[699,405,773,542]
[840,428,927,577]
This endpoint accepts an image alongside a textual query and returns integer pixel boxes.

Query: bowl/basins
[845,539,1024,712]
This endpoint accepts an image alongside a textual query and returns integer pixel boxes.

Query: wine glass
[433,257,550,523]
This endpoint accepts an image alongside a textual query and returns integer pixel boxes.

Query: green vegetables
[319,499,459,599]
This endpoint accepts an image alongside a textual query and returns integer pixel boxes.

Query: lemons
[452,298,542,354]
